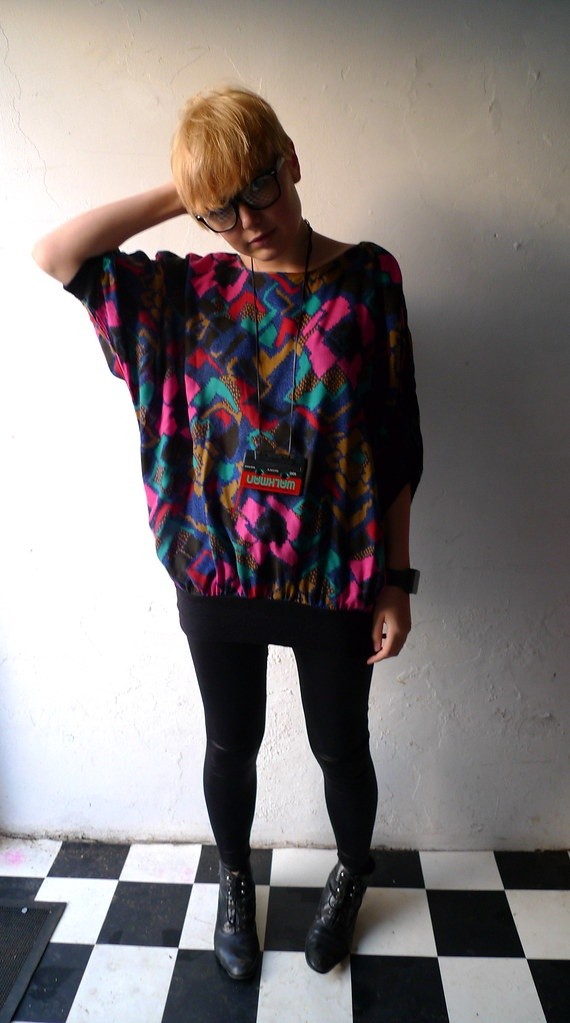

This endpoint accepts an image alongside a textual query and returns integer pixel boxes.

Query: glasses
[195,154,281,233]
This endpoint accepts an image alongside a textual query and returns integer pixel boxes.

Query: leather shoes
[212,861,260,980]
[305,860,367,973]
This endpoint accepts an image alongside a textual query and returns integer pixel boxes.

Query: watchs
[383,568,421,595]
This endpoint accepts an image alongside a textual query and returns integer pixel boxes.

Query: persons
[31,93,422,985]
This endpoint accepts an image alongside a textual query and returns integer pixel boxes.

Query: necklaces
[236,233,316,496]
